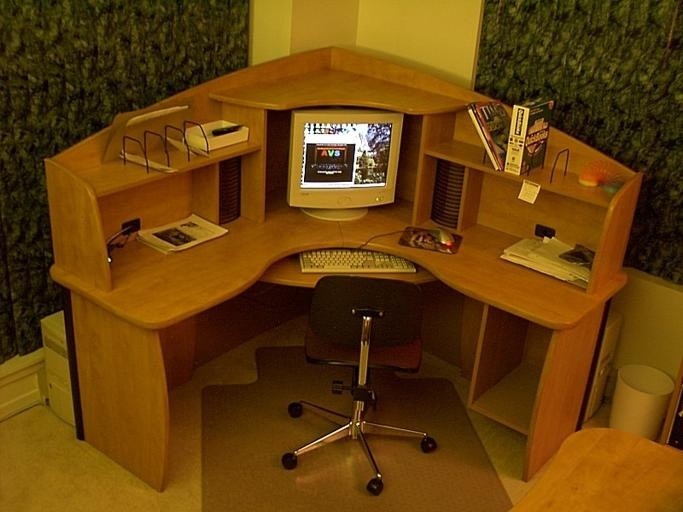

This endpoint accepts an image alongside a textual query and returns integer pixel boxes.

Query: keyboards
[298,247,418,276]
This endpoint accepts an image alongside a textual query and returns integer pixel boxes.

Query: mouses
[429,226,457,246]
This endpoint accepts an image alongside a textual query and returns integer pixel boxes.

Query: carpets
[199,344,514,511]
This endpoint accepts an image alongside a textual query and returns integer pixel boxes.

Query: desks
[43,43,643,493]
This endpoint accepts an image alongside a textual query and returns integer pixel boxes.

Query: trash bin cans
[608,364,675,442]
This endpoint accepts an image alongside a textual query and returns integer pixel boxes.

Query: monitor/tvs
[285,109,404,223]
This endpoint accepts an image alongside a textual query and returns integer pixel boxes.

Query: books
[504,95,554,176]
[466,98,511,171]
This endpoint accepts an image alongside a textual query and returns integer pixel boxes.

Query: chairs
[281,272,438,497]
